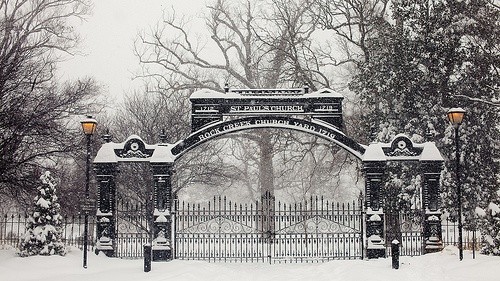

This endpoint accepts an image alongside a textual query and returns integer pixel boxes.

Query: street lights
[79,115,98,268]
[448,106,468,262]
